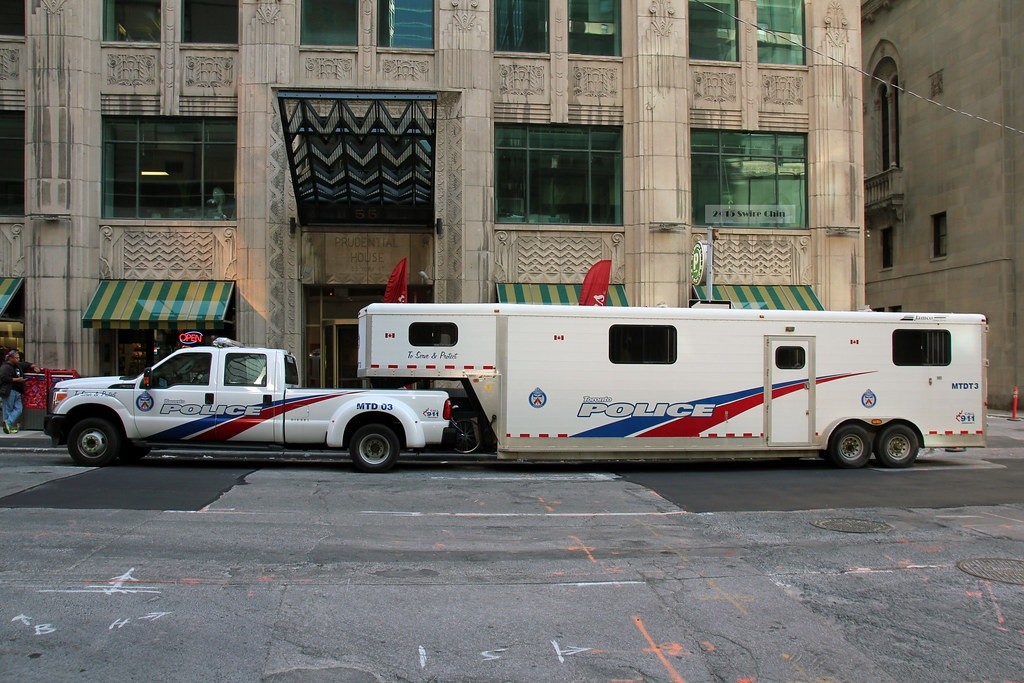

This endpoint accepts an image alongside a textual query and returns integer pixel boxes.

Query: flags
[578,259,610,305]
[382,258,408,302]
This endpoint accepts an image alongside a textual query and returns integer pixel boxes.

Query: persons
[190,358,210,384]
[206,187,235,217]
[0,350,41,433]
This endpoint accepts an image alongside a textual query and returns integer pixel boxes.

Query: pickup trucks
[41,335,452,474]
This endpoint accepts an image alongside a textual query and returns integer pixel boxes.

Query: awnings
[0,276,26,319]
[496,281,629,307]
[693,285,826,310]
[82,279,236,329]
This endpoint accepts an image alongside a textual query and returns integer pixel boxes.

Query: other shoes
[2,420,18,434]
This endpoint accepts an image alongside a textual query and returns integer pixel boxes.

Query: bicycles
[450,404,482,454]
